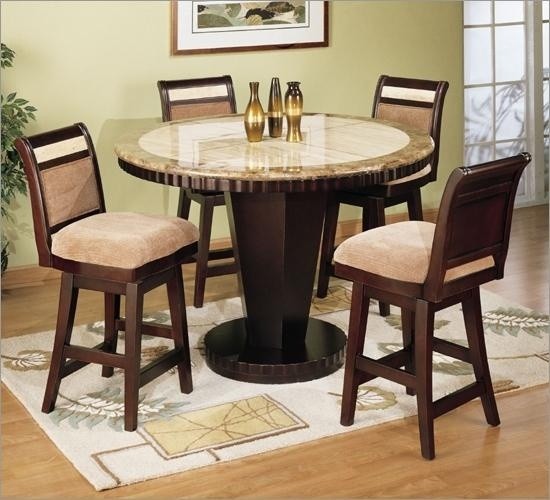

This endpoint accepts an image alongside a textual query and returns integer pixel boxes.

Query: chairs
[157,75,238,308]
[334,152,532,460]
[14,122,201,431]
[316,74,449,317]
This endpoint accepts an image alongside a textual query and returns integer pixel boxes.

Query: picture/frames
[170,0,329,55]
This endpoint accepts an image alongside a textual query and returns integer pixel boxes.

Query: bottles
[244,77,304,142]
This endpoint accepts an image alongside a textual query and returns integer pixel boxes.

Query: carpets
[1,279,550,492]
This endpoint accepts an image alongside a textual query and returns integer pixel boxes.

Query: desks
[113,112,435,385]
